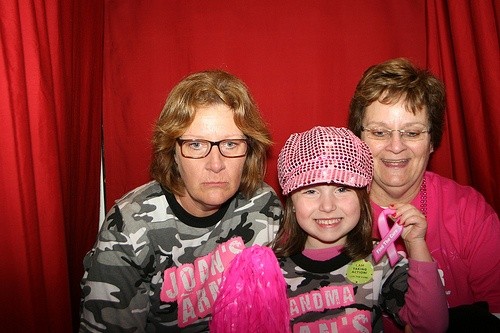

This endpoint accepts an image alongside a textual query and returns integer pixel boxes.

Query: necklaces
[419,172,428,220]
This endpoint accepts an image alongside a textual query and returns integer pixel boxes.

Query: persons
[265,126,449,332]
[77,69,286,333]
[344,57,500,333]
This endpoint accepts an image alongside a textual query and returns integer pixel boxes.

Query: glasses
[174,134,253,161]
[361,124,434,141]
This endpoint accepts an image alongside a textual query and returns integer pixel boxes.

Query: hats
[278,127,374,198]
